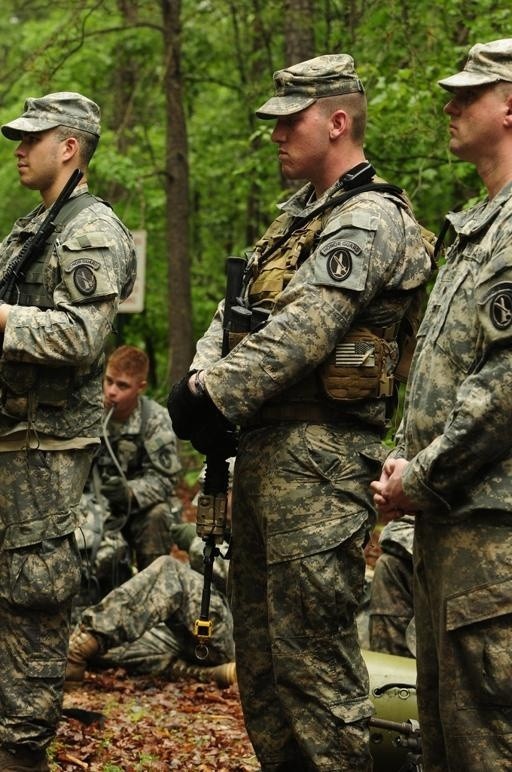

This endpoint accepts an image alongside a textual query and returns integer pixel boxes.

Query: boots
[65,624,100,681]
[177,660,237,688]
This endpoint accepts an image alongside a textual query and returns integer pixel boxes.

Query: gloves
[168,369,236,454]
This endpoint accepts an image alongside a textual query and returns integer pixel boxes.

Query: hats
[438,39,512,93]
[1,92,101,140]
[256,53,364,119]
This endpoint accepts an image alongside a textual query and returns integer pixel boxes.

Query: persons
[167,54,432,772]
[370,37,512,772]
[66,555,238,691]
[188,456,236,587]
[88,345,182,572]
[0,91,138,771]
[370,513,416,658]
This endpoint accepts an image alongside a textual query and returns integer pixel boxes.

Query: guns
[0,168,83,303]
[193,257,249,642]
